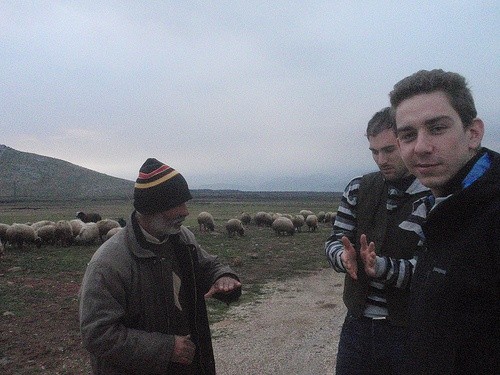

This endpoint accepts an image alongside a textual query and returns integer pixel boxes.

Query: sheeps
[197,211,215,232]
[0,210,127,250]
[240,208,251,225]
[253,209,337,238]
[225,218,245,238]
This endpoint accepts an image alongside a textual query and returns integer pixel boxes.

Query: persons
[325,69,500,375]
[80,158,242,375]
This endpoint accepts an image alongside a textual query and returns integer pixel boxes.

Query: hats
[132,158,193,215]
